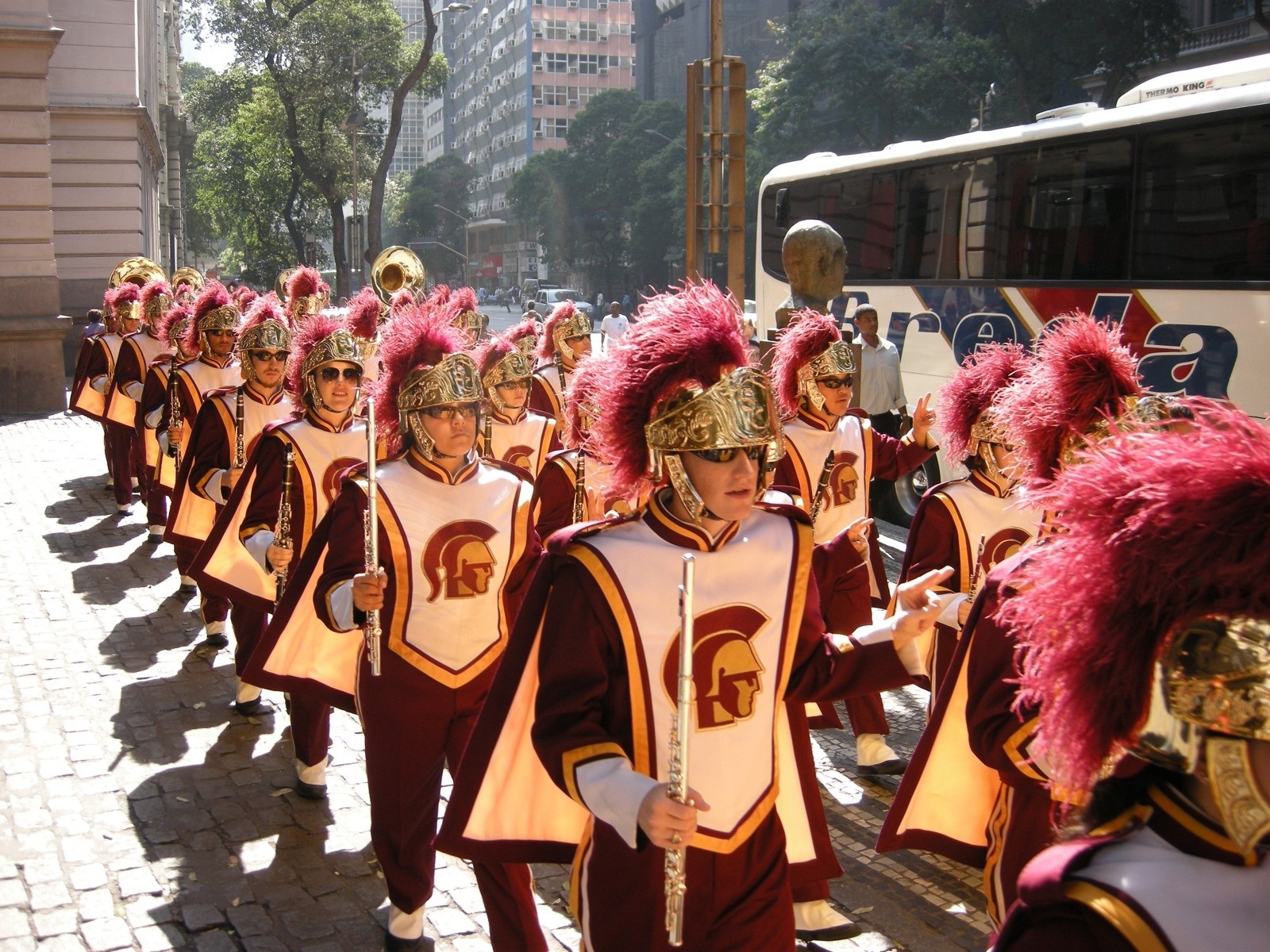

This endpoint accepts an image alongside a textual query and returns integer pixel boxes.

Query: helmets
[105,264,856,499]
[943,310,1204,489]
[988,395,1269,852]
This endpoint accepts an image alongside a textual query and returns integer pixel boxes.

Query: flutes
[482,403,493,459]
[170,355,184,474]
[236,383,246,469]
[810,448,836,524]
[555,349,568,409]
[663,550,695,950]
[362,394,383,677]
[272,440,299,614]
[961,532,987,633]
[574,447,586,521]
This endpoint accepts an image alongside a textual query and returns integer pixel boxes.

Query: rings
[673,830,681,843]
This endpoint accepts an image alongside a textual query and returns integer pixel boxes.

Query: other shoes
[295,777,327,798]
[119,510,133,515]
[792,922,862,940]
[206,634,229,646]
[386,927,423,952]
[235,698,261,715]
[180,584,197,593]
[857,757,908,774]
[105,484,114,490]
[148,534,163,543]
[133,486,140,493]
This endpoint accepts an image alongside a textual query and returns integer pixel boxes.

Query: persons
[775,221,848,329]
[887,305,1270,952]
[66,260,942,952]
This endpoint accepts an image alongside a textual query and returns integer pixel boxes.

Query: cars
[523,288,594,330]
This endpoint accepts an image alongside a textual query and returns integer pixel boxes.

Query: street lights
[433,203,472,286]
[351,2,473,275]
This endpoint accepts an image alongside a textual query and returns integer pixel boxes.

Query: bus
[752,46,1270,531]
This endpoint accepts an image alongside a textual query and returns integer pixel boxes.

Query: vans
[520,279,566,314]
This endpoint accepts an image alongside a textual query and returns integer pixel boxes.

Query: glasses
[209,329,235,336]
[816,377,853,389]
[252,351,291,361]
[496,379,531,389]
[419,405,477,419]
[322,367,359,383]
[571,335,591,341]
[688,446,762,462]
